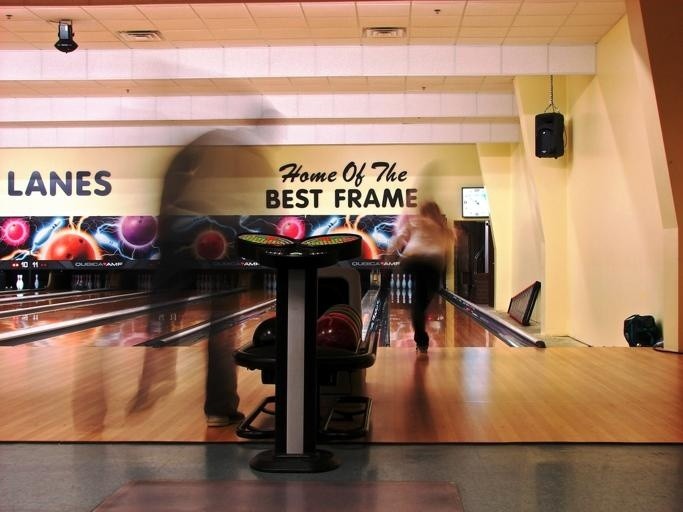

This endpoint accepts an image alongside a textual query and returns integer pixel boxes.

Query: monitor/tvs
[462,187,490,218]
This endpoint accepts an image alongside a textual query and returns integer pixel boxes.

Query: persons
[388,203,455,362]
[127,100,291,428]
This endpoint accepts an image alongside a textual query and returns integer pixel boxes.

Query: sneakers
[205,411,247,429]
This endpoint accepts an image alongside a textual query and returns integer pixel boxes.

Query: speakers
[535,113,564,158]
